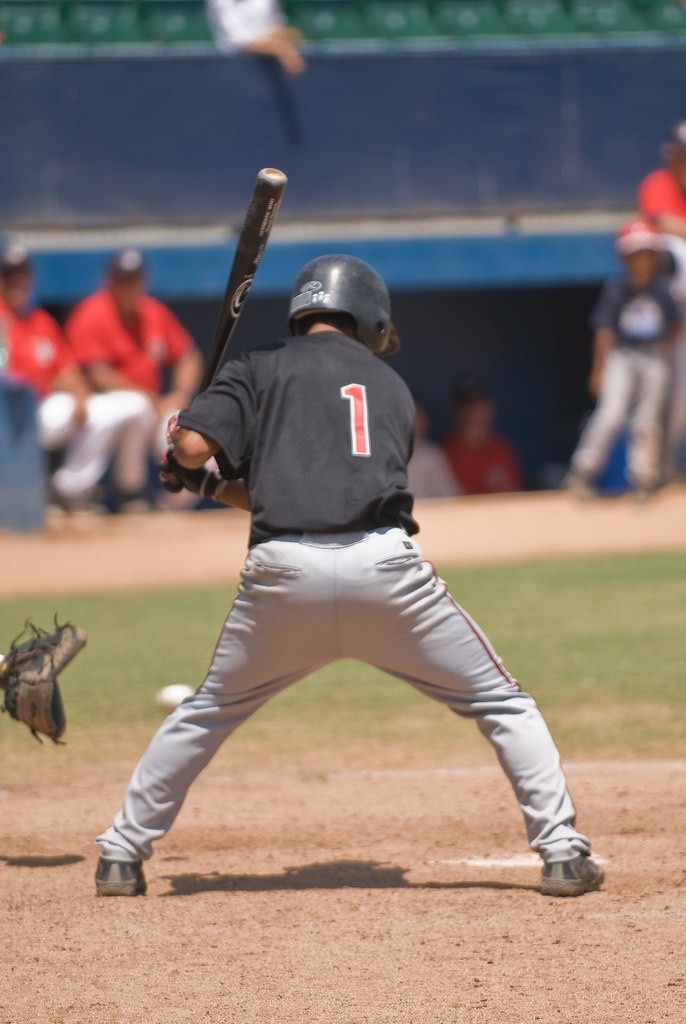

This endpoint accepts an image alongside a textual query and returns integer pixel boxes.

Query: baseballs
[158,683,193,716]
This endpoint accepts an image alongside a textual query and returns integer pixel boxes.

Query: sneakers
[539,853,605,897]
[94,856,147,897]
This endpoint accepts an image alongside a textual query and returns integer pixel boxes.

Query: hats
[111,248,144,274]
[0,245,29,269]
[613,221,662,258]
[669,121,686,145]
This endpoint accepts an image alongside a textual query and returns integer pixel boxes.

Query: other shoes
[560,472,598,501]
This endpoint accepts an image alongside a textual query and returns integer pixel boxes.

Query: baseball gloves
[0,610,89,747]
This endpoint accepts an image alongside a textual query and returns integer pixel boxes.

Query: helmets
[287,254,401,356]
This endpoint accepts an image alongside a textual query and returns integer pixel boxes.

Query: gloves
[158,447,205,493]
[166,408,229,503]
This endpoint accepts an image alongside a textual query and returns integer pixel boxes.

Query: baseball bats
[162,166,289,493]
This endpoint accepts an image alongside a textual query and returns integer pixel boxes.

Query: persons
[205,0,307,75]
[562,220,686,492]
[95,253,606,897]
[406,406,460,498]
[1,250,152,513]
[63,248,202,509]
[637,122,686,235]
[0,612,88,745]
[441,398,518,495]
